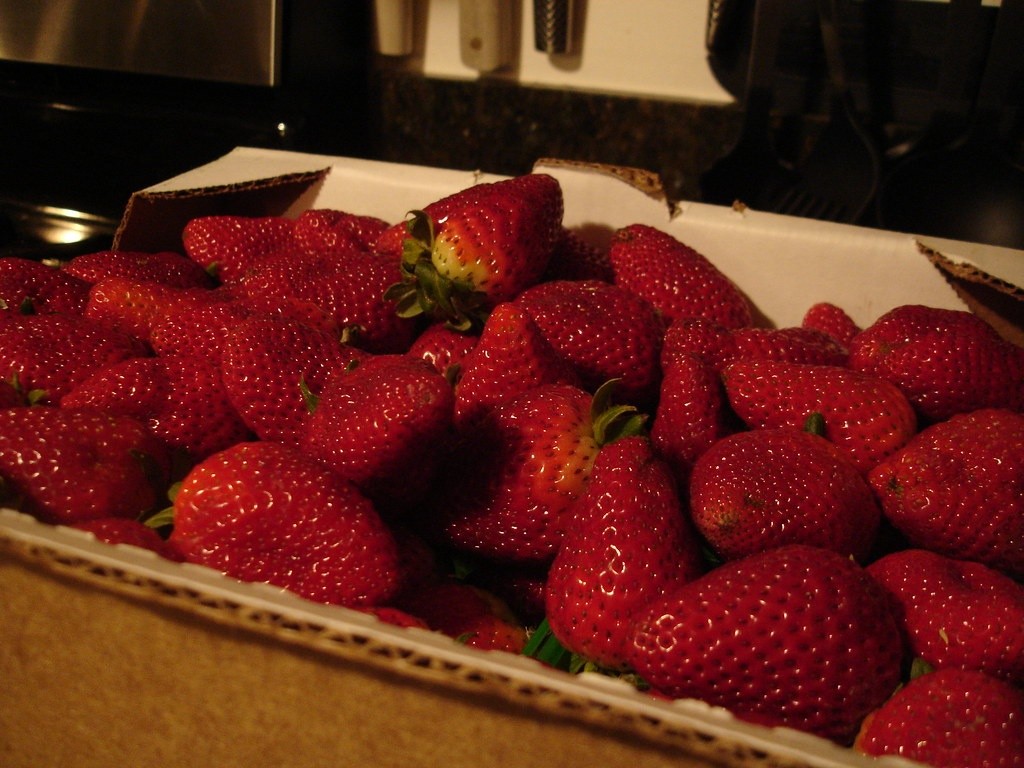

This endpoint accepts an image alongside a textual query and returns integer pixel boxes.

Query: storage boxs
[1,142,1024,768]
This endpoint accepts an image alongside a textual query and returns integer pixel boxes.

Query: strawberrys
[0,171,1024,768]
